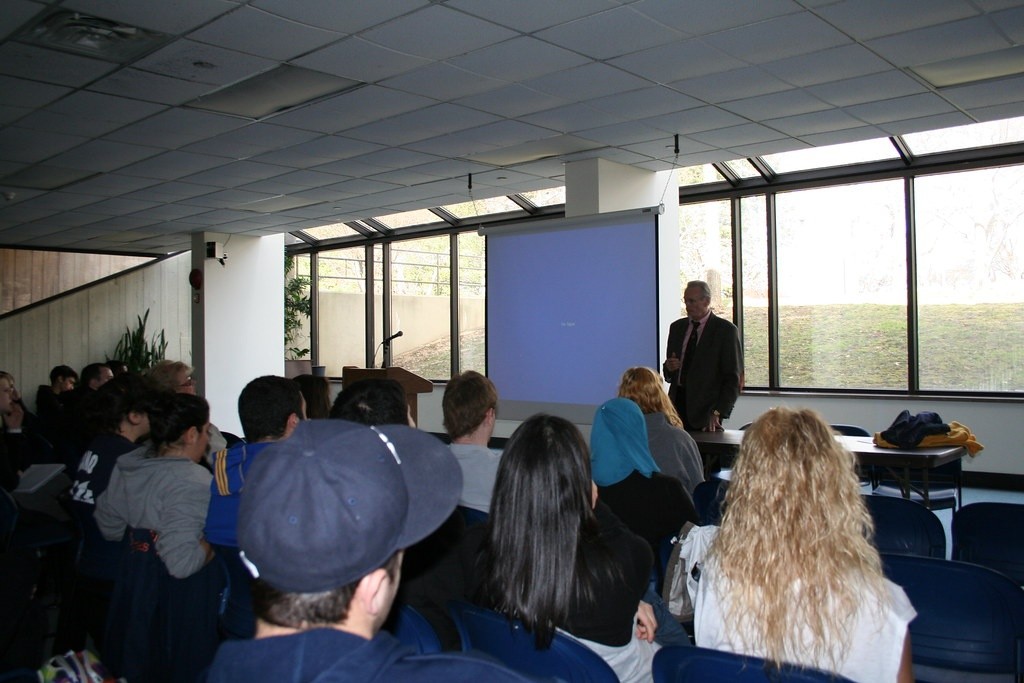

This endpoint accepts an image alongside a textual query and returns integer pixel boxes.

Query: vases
[312,366,325,377]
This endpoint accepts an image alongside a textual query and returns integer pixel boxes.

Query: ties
[679,320,700,388]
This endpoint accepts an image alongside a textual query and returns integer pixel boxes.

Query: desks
[419,419,967,510]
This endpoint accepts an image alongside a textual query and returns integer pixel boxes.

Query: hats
[234,415,462,592]
[91,372,171,416]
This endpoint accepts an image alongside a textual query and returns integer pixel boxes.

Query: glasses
[176,377,192,388]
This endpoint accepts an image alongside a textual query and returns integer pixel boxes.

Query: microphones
[381,331,403,344]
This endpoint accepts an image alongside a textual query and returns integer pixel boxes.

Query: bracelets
[711,409,720,416]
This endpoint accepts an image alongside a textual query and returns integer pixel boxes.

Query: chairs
[829,425,879,494]
[1,470,1023,683]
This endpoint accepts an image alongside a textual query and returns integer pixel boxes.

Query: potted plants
[285,255,312,378]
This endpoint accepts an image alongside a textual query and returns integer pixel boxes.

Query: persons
[691,404,919,683]
[204,420,523,682]
[292,373,331,420]
[329,377,417,432]
[473,412,692,682]
[662,280,745,436]
[588,398,697,565]
[1,360,227,580]
[439,370,509,512]
[205,376,310,549]
[614,365,705,502]
